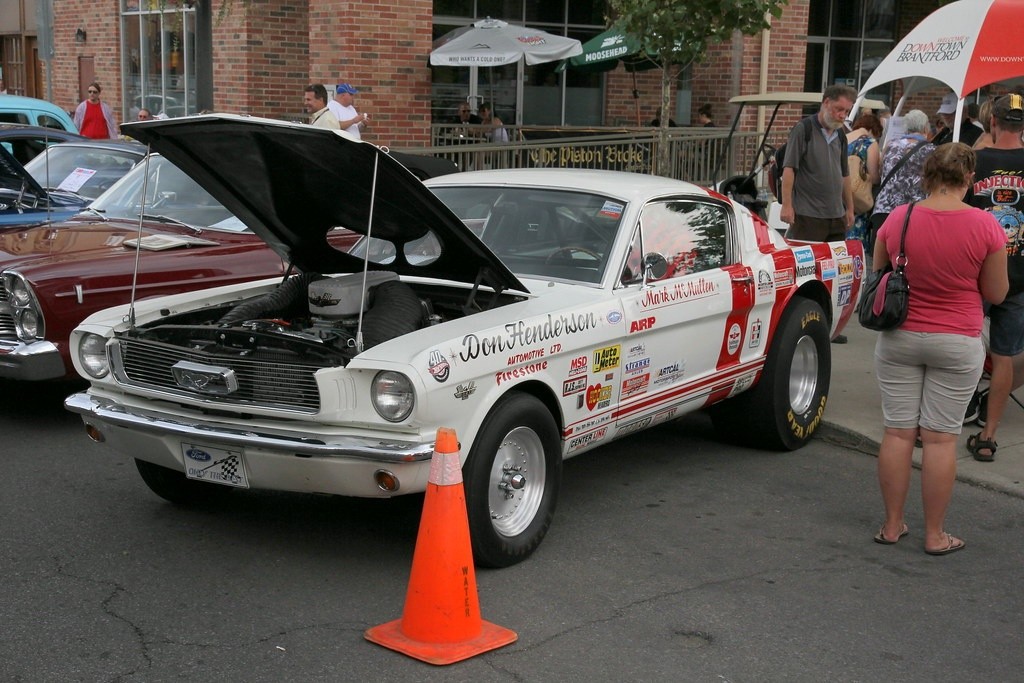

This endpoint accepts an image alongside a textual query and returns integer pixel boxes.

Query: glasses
[459,109,471,112]
[88,91,100,93]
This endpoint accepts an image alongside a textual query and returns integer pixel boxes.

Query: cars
[64,112,867,570]
[0,153,492,380]
[133,93,196,118]
[0,93,198,228]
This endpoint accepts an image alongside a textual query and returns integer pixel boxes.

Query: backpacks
[771,117,810,203]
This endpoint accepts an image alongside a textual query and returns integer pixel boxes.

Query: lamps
[75,28,86,42]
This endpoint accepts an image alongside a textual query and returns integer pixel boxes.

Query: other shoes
[964,390,982,422]
[979,389,992,426]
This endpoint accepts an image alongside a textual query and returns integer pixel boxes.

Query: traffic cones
[362,428,519,664]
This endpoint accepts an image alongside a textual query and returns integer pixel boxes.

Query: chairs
[628,212,703,282]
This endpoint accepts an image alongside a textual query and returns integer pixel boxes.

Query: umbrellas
[555,22,682,127]
[429,17,583,140]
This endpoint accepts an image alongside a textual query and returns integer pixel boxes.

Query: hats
[937,92,967,113]
[336,83,357,94]
[992,93,1024,121]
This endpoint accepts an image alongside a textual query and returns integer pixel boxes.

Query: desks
[435,135,488,142]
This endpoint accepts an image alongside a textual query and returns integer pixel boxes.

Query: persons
[441,101,483,166]
[462,102,508,171]
[968,103,986,132]
[74,83,117,139]
[963,308,992,427]
[138,109,151,121]
[327,83,370,172]
[649,106,678,127]
[972,100,994,150]
[872,141,1010,555]
[967,94,1024,462]
[932,93,984,201]
[304,84,341,172]
[869,109,937,275]
[844,114,884,254]
[677,103,717,182]
[780,82,858,243]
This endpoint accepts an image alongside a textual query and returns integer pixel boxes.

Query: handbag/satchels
[858,201,914,330]
[843,134,874,211]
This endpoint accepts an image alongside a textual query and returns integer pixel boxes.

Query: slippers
[874,523,908,543]
[928,532,965,554]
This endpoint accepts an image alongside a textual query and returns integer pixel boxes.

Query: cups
[463,122,469,135]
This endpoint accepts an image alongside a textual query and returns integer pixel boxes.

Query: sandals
[966,431,997,461]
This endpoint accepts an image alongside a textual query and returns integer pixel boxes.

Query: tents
[848,0,1024,143]
